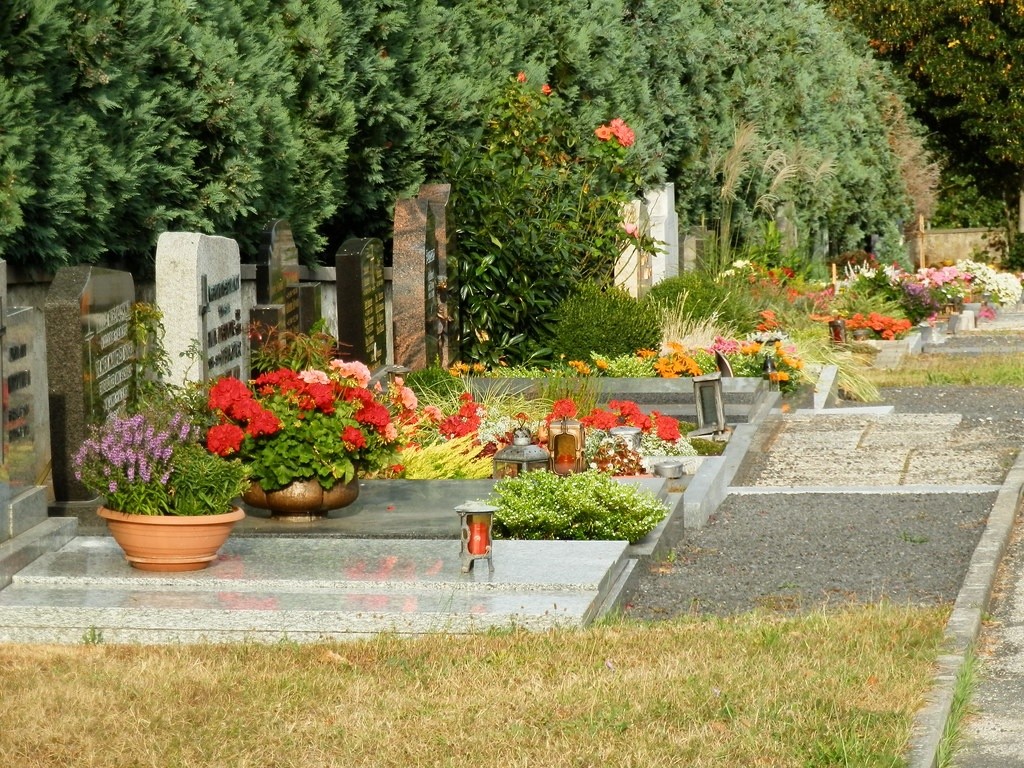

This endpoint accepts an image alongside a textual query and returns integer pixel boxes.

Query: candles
[468,521,490,556]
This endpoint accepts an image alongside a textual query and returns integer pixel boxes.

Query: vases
[98,502,244,569]
[241,469,363,520]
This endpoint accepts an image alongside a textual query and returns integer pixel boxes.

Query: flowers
[72,74,1018,522]
